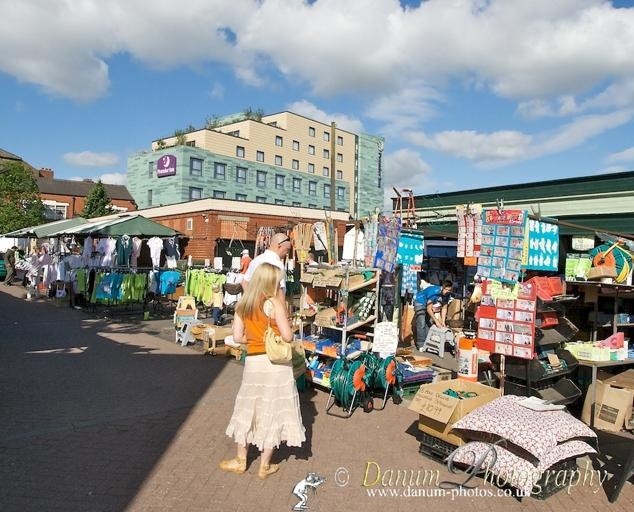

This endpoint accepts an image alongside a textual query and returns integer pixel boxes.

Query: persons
[2,246,18,287]
[488,226,494,234]
[487,248,492,256]
[419,279,452,350]
[25,243,51,300]
[238,249,252,273]
[482,297,532,345]
[219,263,308,478]
[240,232,293,300]
[70,244,80,255]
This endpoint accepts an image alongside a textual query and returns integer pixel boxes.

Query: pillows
[444,439,599,497]
[450,394,597,464]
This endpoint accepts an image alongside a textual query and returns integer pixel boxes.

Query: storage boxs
[203,328,234,358]
[407,379,501,447]
[427,365,452,381]
[224,344,243,361]
[409,356,433,368]
[582,370,634,432]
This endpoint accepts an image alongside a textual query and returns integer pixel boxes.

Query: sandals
[260,464,279,480]
[220,457,248,475]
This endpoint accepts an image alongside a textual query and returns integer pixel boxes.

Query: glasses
[279,236,291,245]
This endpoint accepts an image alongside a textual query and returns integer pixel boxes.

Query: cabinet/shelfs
[565,281,634,344]
[299,265,380,391]
[503,301,583,406]
[565,342,628,362]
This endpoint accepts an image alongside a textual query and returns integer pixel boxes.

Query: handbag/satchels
[263,303,293,365]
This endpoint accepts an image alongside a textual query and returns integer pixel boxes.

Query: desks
[577,360,634,432]
